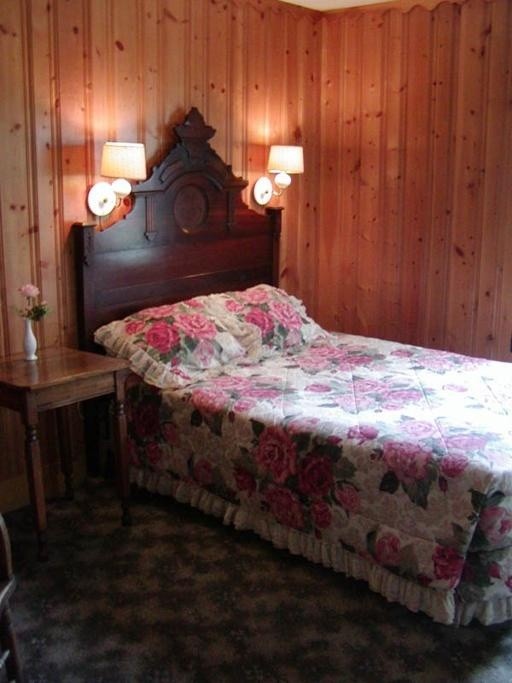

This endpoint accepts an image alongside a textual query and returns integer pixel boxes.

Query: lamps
[251,143,305,206]
[88,137,148,217]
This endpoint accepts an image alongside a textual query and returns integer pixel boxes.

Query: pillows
[92,281,333,390]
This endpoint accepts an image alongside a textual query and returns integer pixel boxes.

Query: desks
[1,343,151,562]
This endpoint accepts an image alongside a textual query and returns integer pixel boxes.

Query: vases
[22,318,38,363]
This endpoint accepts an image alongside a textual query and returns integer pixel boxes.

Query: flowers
[7,281,51,325]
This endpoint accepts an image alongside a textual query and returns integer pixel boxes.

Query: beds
[71,105,511,624]
